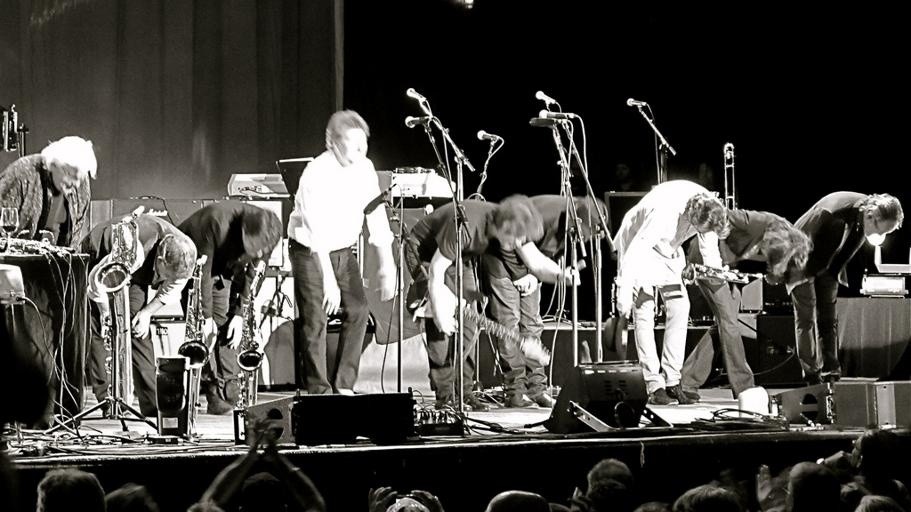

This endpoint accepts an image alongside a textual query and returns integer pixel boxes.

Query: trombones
[237,289,262,416]
[96,218,138,423]
[178,256,210,440]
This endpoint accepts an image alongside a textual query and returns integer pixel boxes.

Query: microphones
[364,186,393,214]
[723,142,735,160]
[536,91,554,105]
[626,97,646,107]
[477,130,500,141]
[406,87,426,103]
[538,109,574,122]
[405,115,429,128]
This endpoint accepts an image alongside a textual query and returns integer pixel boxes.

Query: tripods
[34,300,159,442]
[429,158,504,433]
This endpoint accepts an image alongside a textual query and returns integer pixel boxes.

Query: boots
[207,402,237,415]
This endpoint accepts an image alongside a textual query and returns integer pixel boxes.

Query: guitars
[406,261,551,367]
[683,261,750,284]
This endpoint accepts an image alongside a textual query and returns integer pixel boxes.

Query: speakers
[244,393,415,449]
[777,379,911,427]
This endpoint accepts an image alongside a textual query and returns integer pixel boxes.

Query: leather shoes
[505,392,556,408]
[649,385,700,405]
[436,396,490,412]
